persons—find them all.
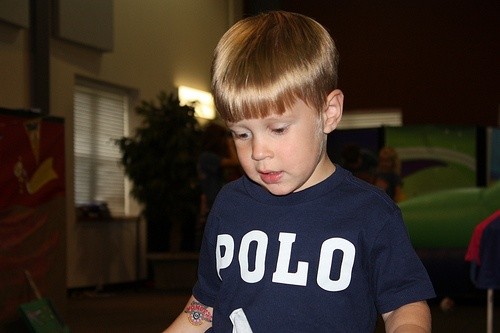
[339,141,404,203]
[441,210,500,313]
[162,11,437,333]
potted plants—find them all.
[112,91,231,289]
